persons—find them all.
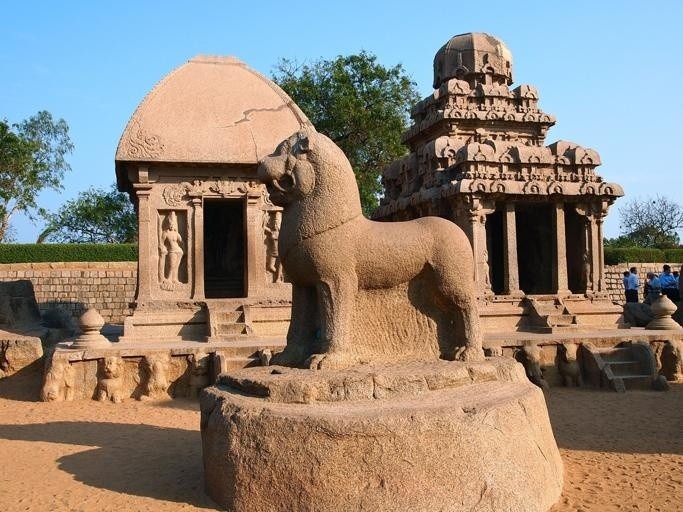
[623,264,683,304]
[159,212,183,281]
[261,211,283,284]
